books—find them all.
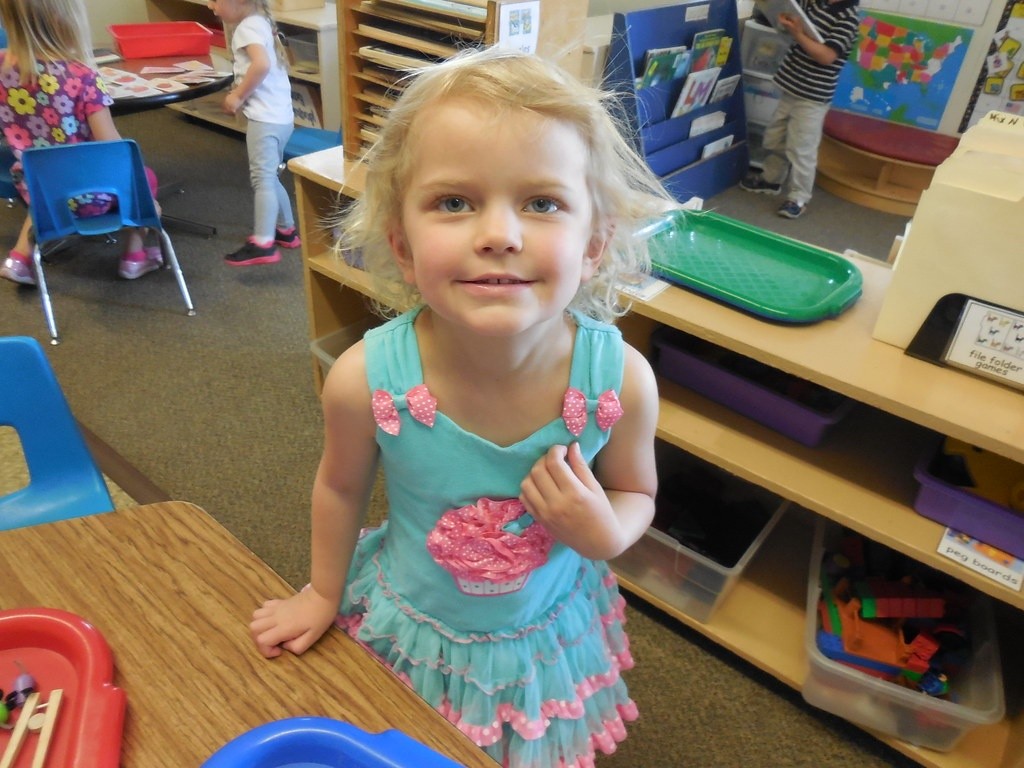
[755,0,826,47]
[352,1,497,166]
[633,29,741,166]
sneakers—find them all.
[224,235,281,266]
[778,200,806,218]
[739,174,782,195]
[0,257,37,285]
[119,247,164,279]
[276,225,301,247]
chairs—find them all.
[24,137,197,345]
[0,336,120,529]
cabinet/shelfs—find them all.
[145,1,339,157]
[288,0,1024,767]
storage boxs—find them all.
[801,513,1008,752]
[613,482,792,626]
[310,316,385,388]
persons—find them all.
[206,0,301,266]
[739,1,861,219]
[0,0,166,284]
[250,51,659,768]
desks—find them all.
[39,48,237,265]
[1,500,501,765]
